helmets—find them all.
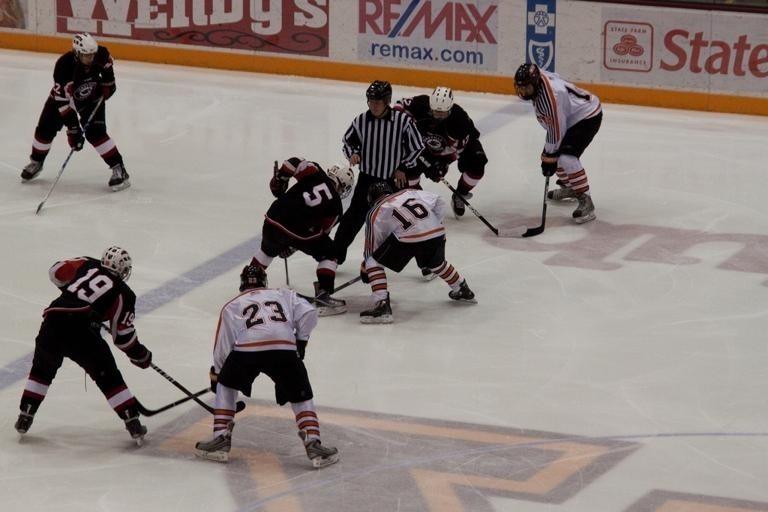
[239,266,267,291]
[429,86,454,121]
[366,80,391,104]
[514,63,540,101]
[327,166,355,198]
[366,182,393,207]
[100,246,131,281]
[72,33,98,54]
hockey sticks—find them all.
[522,172,549,237]
[274,160,314,303]
[103,324,246,416]
[131,386,212,417]
[36,94,104,213]
[419,157,527,238]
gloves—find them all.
[67,131,84,151]
[103,84,116,99]
[541,151,560,176]
[360,260,369,284]
[209,365,219,393]
[426,159,448,182]
[270,176,288,196]
[130,348,152,369]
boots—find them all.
[15,403,36,433]
[448,277,475,300]
[313,281,346,307]
[109,157,129,186]
[418,262,432,276]
[196,421,236,451]
[360,291,392,316]
[119,407,147,438]
[21,154,44,179]
[572,188,595,218]
[297,427,337,459]
[452,185,472,216]
[548,179,577,200]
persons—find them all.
[249,157,354,317]
[332,80,440,281]
[21,33,130,186]
[195,265,337,459]
[393,86,489,216]
[360,182,475,318]
[14,245,152,439]
[513,63,603,219]
